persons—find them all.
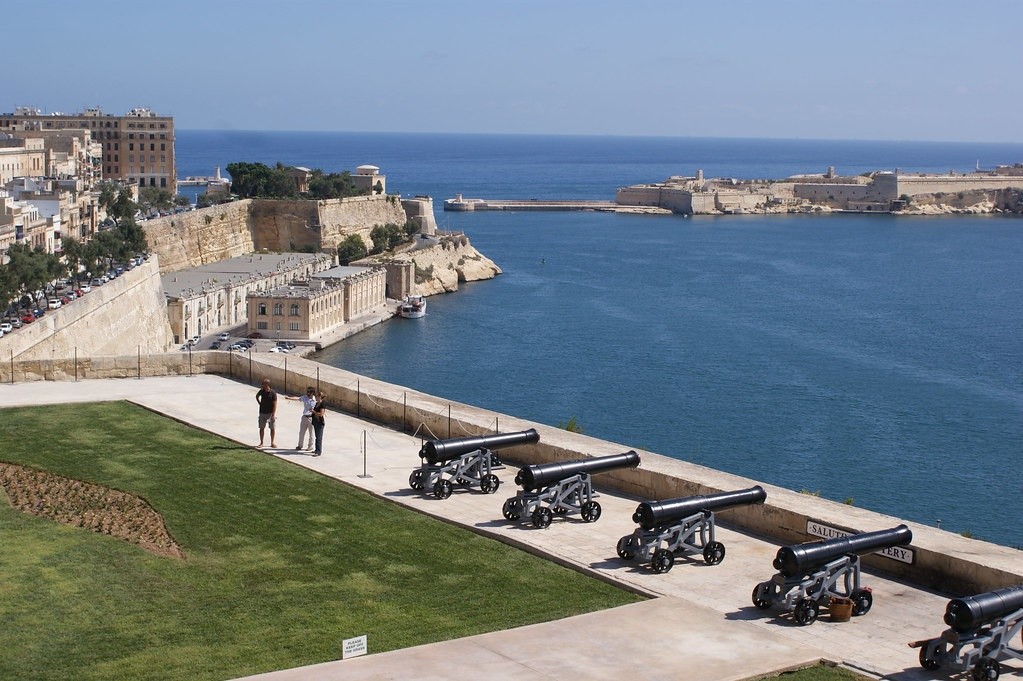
[256,379,278,448]
[285,387,326,457]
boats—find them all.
[400,292,428,318]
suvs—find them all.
[420,232,430,239]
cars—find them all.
[0,249,150,342]
[178,329,297,356]
[131,204,218,225]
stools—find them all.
[829,602,853,621]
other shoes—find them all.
[258,444,263,447]
[305,448,313,451]
[272,444,276,448]
[312,453,319,457]
[296,446,303,450]
[312,451,315,452]
